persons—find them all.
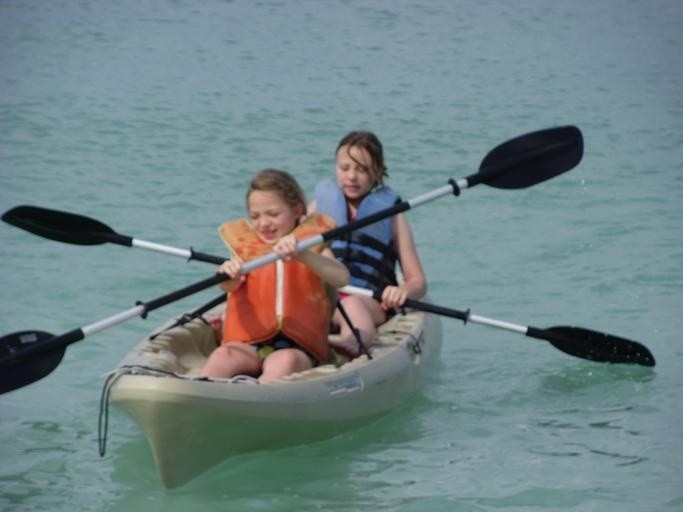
[201,169,350,383]
[307,131,426,358]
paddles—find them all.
[0,124,585,397]
[0,204,657,368]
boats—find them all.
[101,271,431,489]
